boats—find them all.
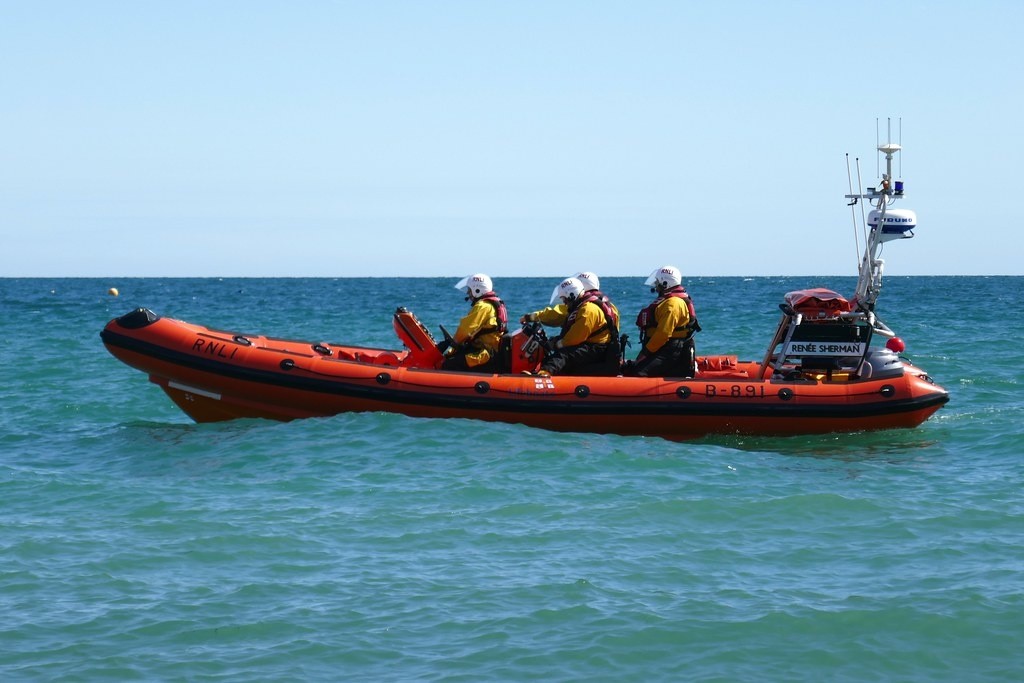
[101,117,950,447]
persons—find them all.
[520,272,620,331]
[439,273,508,372]
[521,278,620,377]
[620,264,698,378]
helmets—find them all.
[559,277,584,301]
[467,273,493,297]
[578,271,600,291]
[656,265,682,289]
